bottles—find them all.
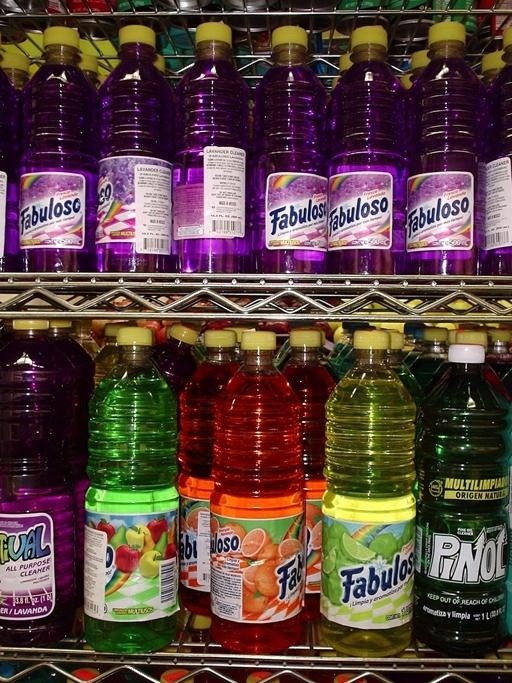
[3,2,512,308]
[0,293,512,678]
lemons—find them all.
[320,519,414,608]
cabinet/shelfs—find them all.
[1,0,511,683]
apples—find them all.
[96,516,177,580]
